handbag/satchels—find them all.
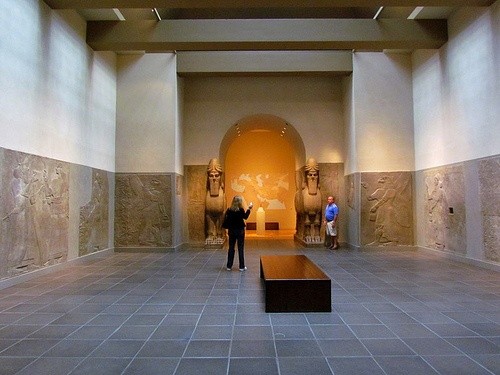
[222,208,229,229]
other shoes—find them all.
[239,267,248,272]
[227,266,233,270]
[329,244,339,250]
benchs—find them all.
[259,255,331,313]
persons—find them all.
[323,195,340,249]
[221,196,253,272]
